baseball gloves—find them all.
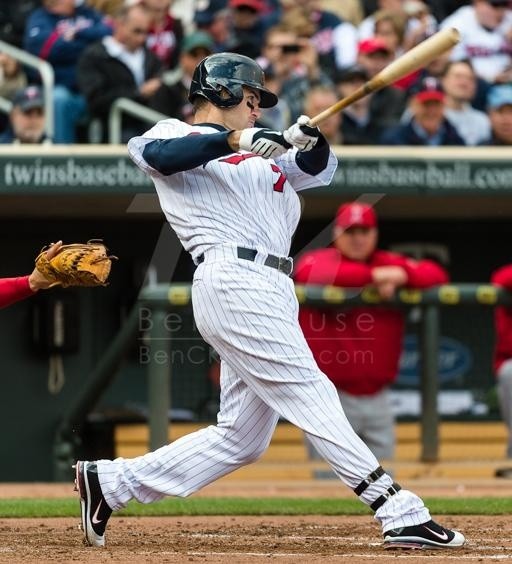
[34,238,118,289]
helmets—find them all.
[188,50,278,111]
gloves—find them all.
[239,126,292,159]
[284,114,320,153]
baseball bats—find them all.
[262,28,460,158]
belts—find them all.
[196,246,294,276]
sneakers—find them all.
[383,521,465,550]
[73,458,113,547]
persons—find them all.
[483,264,512,482]
[293,200,449,485]
[71,50,473,551]
[1,0,512,152]
[0,239,117,308]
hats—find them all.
[335,201,376,229]
[487,82,512,111]
[359,37,395,56]
[15,85,48,111]
[414,76,445,104]
